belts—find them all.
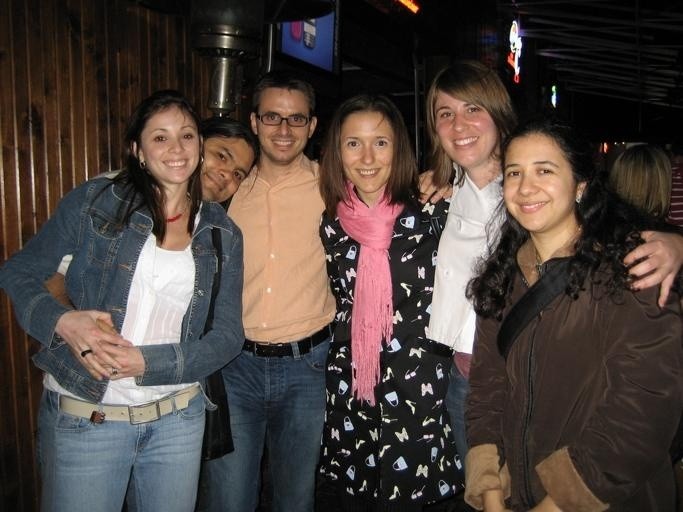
[57,384,201,424]
[243,319,339,357]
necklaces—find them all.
[162,196,191,222]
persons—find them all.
[0,89,243,511]
[463,119,682,511]
[424,60,682,511]
[39,115,261,310]
[608,143,671,220]
[312,90,475,509]
[201,69,456,509]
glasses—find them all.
[255,111,313,128]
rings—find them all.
[80,348,92,358]
[111,366,117,377]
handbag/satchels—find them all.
[200,370,235,462]
